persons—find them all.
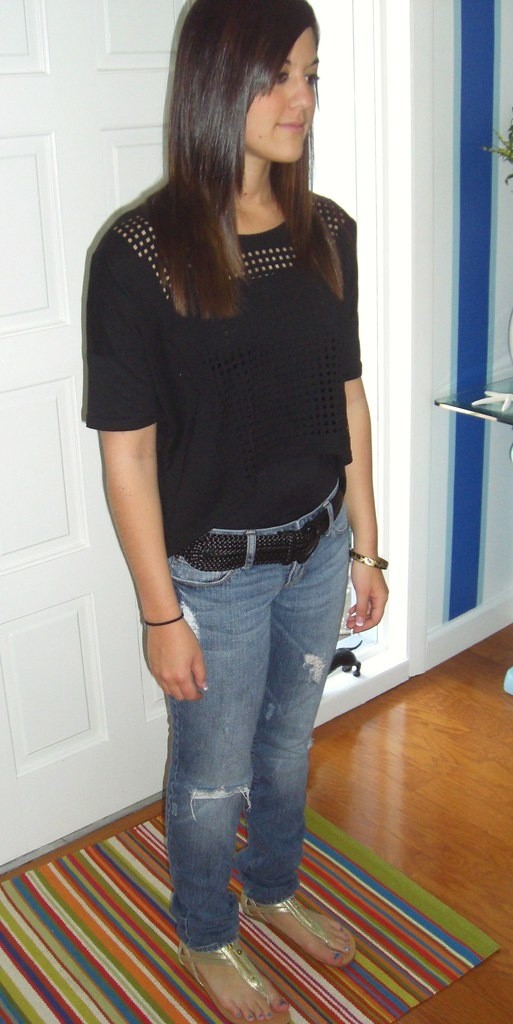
[85,0,389,1024]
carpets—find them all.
[0,799,501,1024]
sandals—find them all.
[178,936,291,1024]
[242,885,356,967]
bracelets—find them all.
[144,612,184,627]
[349,549,388,570]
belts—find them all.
[174,468,348,573]
